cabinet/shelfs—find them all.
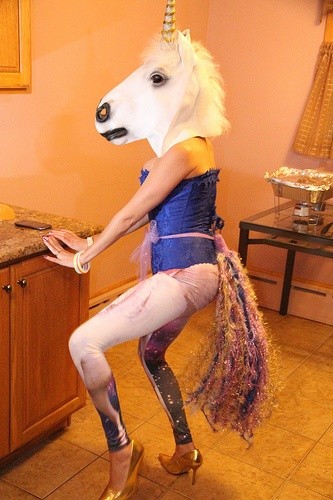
[0,201,105,468]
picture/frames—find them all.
[0,0,32,89]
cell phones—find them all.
[15,220,52,230]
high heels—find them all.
[157,446,205,488]
[94,436,148,500]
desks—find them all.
[237,200,333,315]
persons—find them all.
[43,29,231,500]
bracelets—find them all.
[73,251,90,274]
[87,237,93,246]
